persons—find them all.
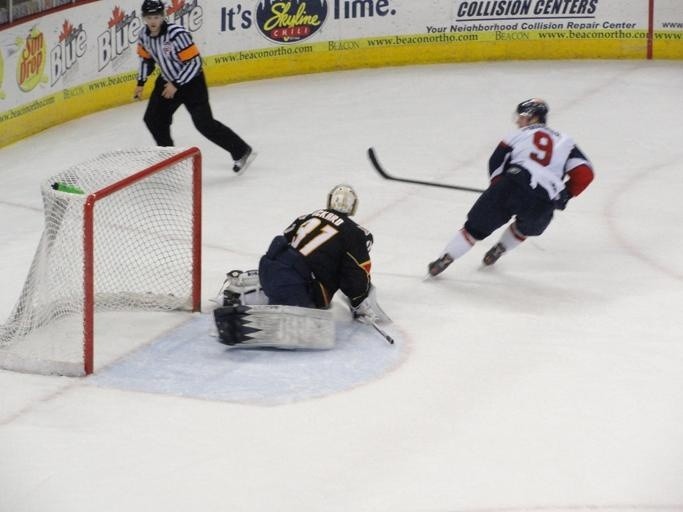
[134,0,252,172]
[428,97,594,276]
[213,182,378,350]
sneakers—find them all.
[232,146,252,173]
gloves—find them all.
[552,187,571,210]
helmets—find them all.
[141,0,164,32]
[326,183,359,217]
[515,97,547,128]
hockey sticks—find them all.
[368,149,487,194]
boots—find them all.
[428,253,453,277]
[483,242,506,265]
